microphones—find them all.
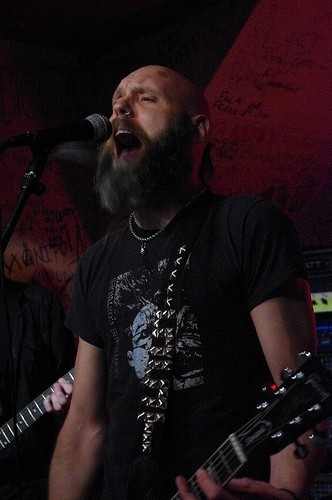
[0,114,113,150]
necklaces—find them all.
[126,187,205,258]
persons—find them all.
[42,63,328,500]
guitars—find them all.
[172,349,331,500]
